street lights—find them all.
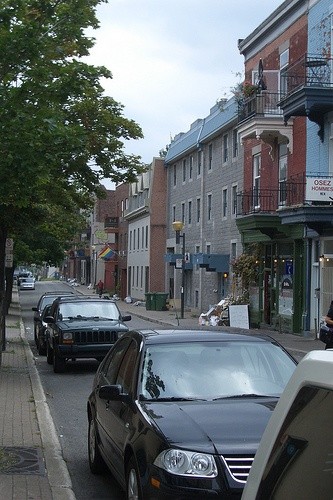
[91,245,97,291]
[172,221,186,319]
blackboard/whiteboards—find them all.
[228,304,250,330]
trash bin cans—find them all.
[144,292,156,311]
[95,284,103,294]
[156,292,169,312]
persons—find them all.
[325,300,333,350]
[98,280,104,296]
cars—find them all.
[13,271,37,291]
[31,291,75,357]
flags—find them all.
[98,246,115,261]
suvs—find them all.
[86,325,299,500]
[239,348,333,500]
[43,294,132,373]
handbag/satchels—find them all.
[319,325,333,345]
